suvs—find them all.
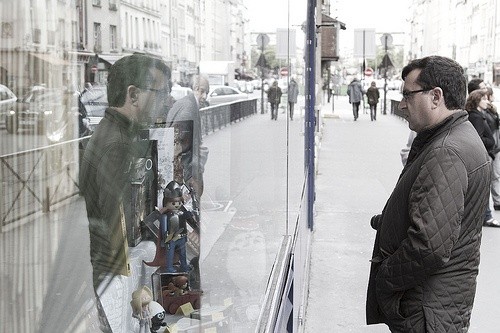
[6,84,88,135]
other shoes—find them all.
[485,218,500,227]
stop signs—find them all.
[365,68,373,77]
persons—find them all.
[347,78,364,121]
[267,80,282,120]
[366,56,492,333]
[80,53,176,332]
[483,87,500,130]
[465,90,500,228]
[366,82,380,121]
[166,75,209,201]
[288,77,299,121]
[468,79,500,211]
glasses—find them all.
[403,87,433,101]
[140,86,168,99]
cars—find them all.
[78,86,111,132]
[0,85,19,126]
[169,83,251,111]
